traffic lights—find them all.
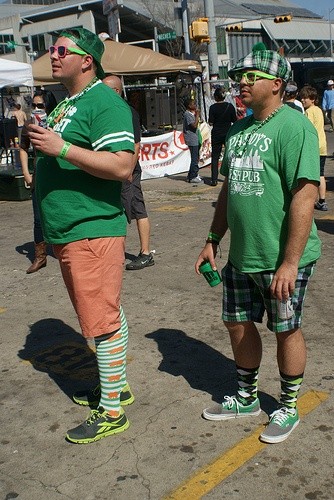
[273,14,292,23]
[189,20,209,40]
[225,22,242,32]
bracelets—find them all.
[205,231,223,246]
[58,142,71,159]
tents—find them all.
[30,34,203,122]
[0,58,34,99]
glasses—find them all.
[233,70,277,86]
[31,103,47,108]
[49,45,87,58]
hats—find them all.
[59,28,106,79]
[227,42,292,82]
[285,84,297,92]
[327,80,334,85]
[213,87,226,102]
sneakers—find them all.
[65,404,129,443]
[203,394,261,421]
[73,381,135,407]
[125,249,156,269]
[259,405,300,444]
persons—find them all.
[19,90,58,274]
[283,78,334,211]
[182,99,204,184]
[0,98,27,145]
[191,42,321,444]
[203,83,252,186]
[26,27,135,444]
[103,75,155,270]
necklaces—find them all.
[55,77,99,123]
[235,104,284,156]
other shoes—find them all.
[314,201,328,211]
[186,173,207,185]
[209,179,219,187]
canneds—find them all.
[30,109,47,130]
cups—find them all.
[199,260,221,288]
[31,130,48,157]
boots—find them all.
[26,241,47,273]
